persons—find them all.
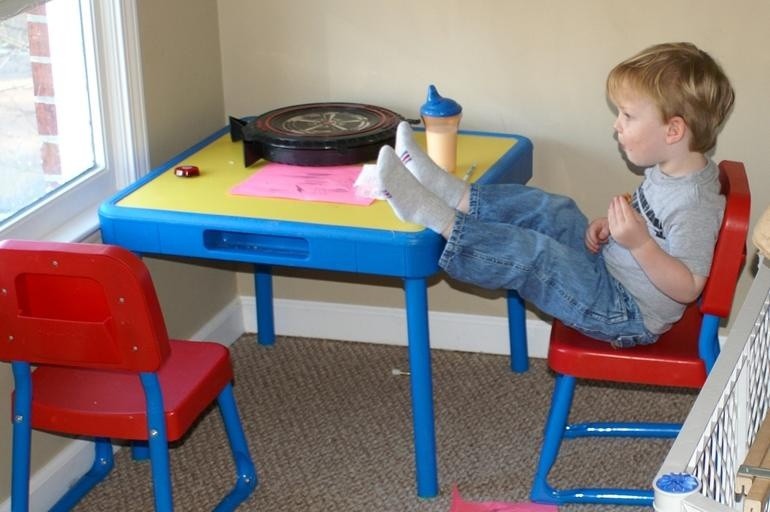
[373,37,738,352]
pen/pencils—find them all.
[463,165,476,182]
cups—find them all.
[419,84,463,173]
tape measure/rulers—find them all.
[175,165,199,177]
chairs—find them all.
[1,240,257,512]
[529,160,752,506]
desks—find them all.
[98,115,534,499]
